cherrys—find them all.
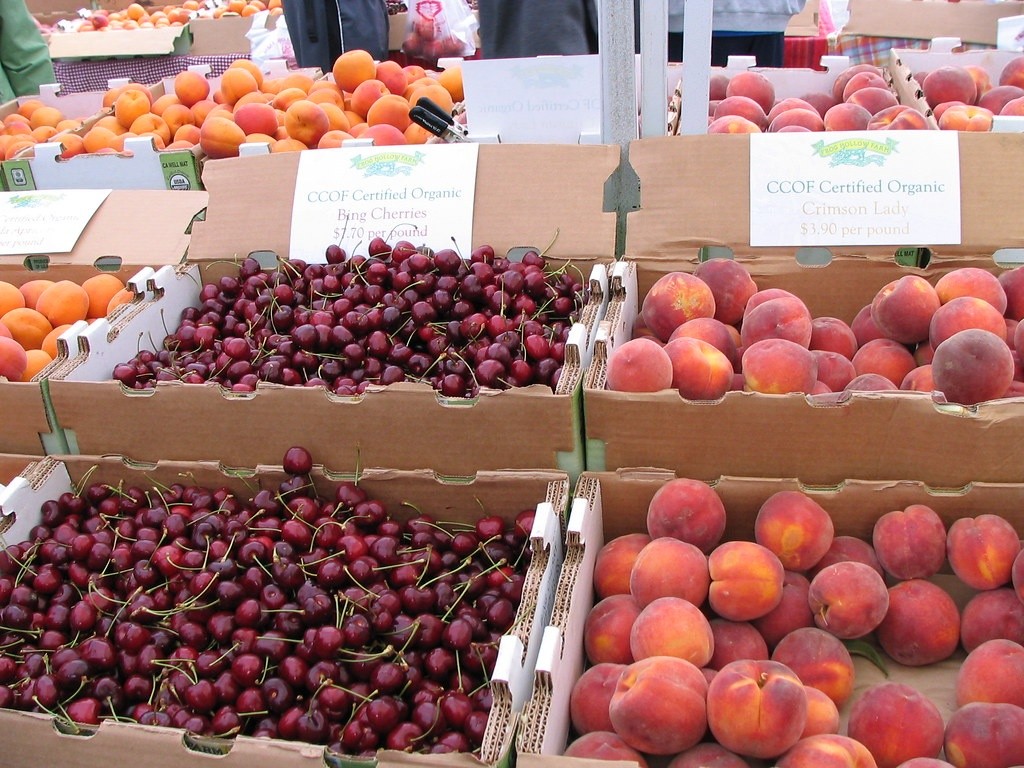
[111,210,588,397]
[0,445,535,760]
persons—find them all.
[475,0,598,59]
[0,0,58,105]
[281,0,390,75]
[667,0,806,68]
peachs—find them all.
[77,0,208,30]
[560,475,1024,768]
[197,0,284,19]
[603,258,1024,404]
[45,49,465,159]
[0,272,134,383]
[0,98,93,162]
[401,17,464,60]
[912,55,1024,132]
[708,63,930,134]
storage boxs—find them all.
[841,0,1024,46]
[1,60,323,191]
[460,54,684,143]
[583,130,1024,490]
[46,144,620,492]
[0,77,133,191]
[882,36,1024,131]
[0,188,208,455]
[0,454,565,768]
[25,1,277,65]
[516,465,1024,768]
